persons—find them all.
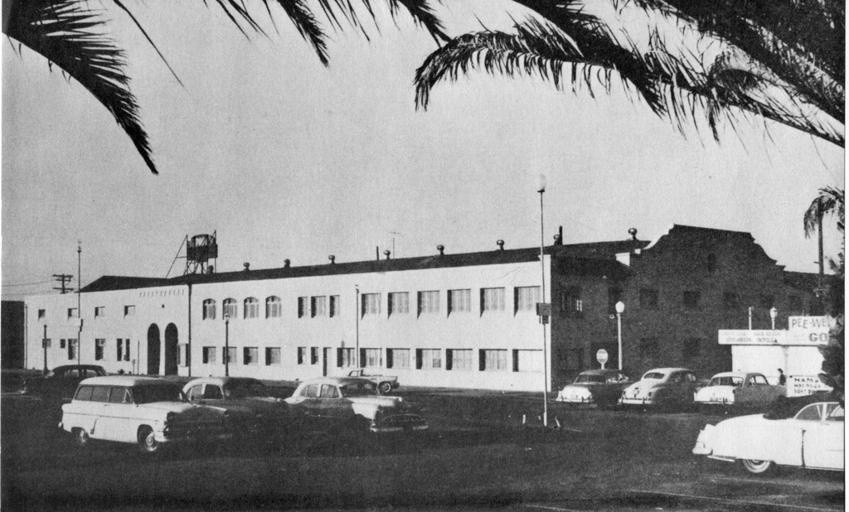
[775,367,786,387]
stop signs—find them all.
[595,348,609,366]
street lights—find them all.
[768,306,779,330]
[73,236,84,367]
[532,169,552,430]
[223,311,230,376]
[614,299,626,370]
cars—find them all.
[615,365,700,414]
[689,398,845,482]
[689,369,790,415]
[551,367,633,411]
